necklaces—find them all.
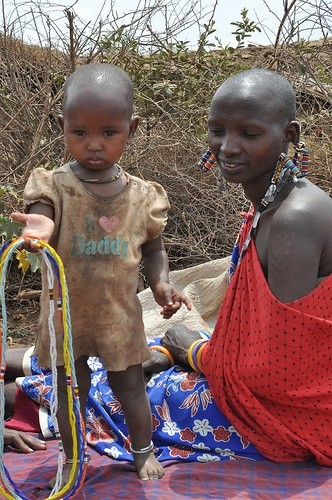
[72,163,125,185]
[235,176,278,267]
[0,232,90,500]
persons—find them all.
[2,426,48,455]
[8,62,193,491]
[0,67,332,470]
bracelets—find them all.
[186,338,210,375]
[198,328,217,343]
[148,344,175,367]
